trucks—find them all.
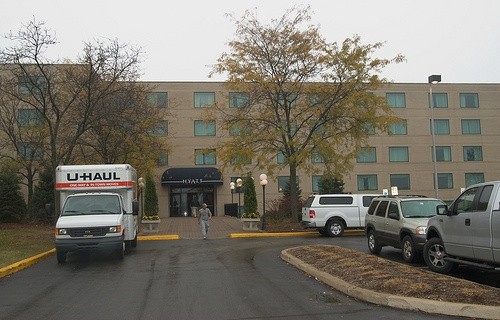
[55,164,138,264]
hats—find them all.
[202,204,207,208]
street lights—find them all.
[429,75,441,199]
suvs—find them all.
[301,193,388,237]
[423,181,500,275]
[365,194,449,263]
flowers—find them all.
[241,213,257,219]
[143,215,159,220]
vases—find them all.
[141,220,161,235]
[242,218,260,231]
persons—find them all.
[190,197,200,218]
[198,203,212,240]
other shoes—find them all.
[204,237,206,239]
